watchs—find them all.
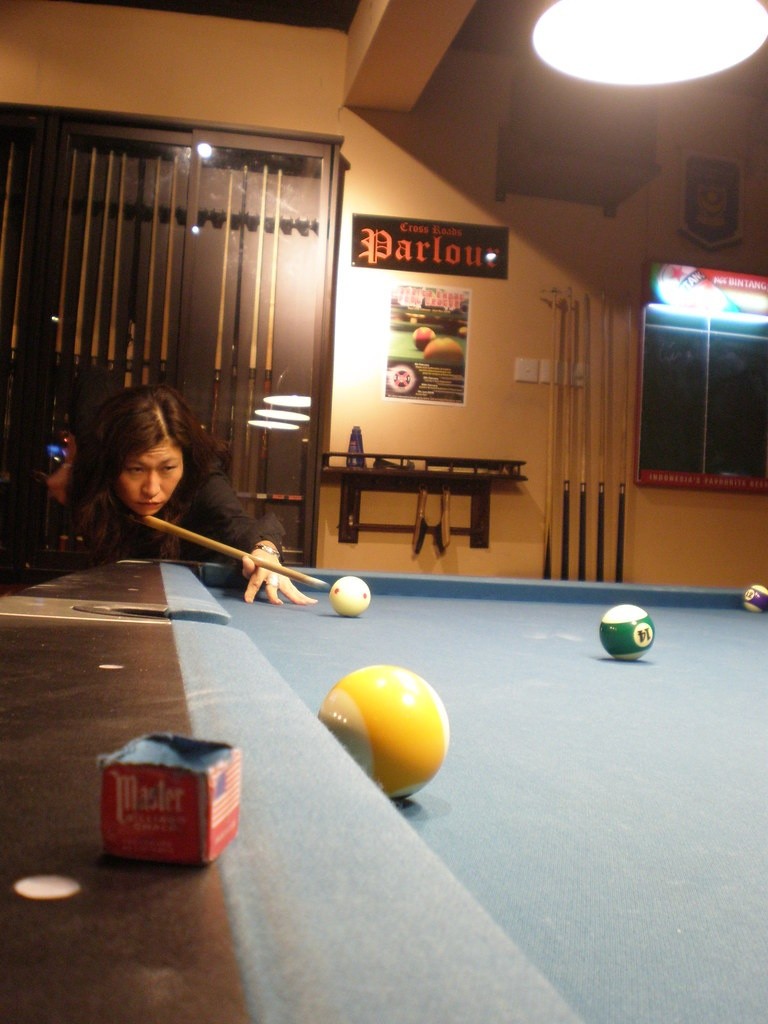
[249,542,280,560]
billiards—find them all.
[312,663,452,802]
[598,602,659,663]
[328,574,373,618]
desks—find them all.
[0,555,768,1024]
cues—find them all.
[540,284,636,582]
[0,138,287,583]
[29,470,332,593]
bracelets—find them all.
[61,464,72,472]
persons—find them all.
[46,383,319,606]
